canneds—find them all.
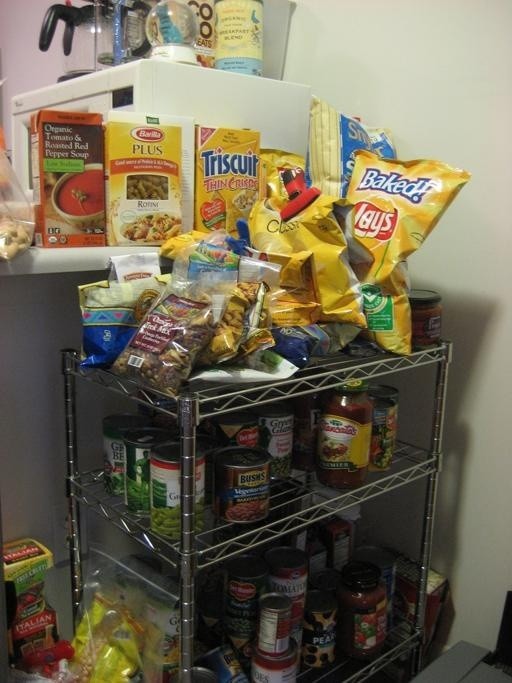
[294,385,322,471]
[368,384,399,471]
[257,409,295,478]
[115,545,387,683]
[214,446,273,523]
[408,288,443,345]
[150,442,205,539]
[318,375,373,488]
[212,416,258,448]
[122,426,172,519]
[104,415,143,498]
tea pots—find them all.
[39,0,157,84]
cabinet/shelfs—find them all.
[59,337,457,683]
